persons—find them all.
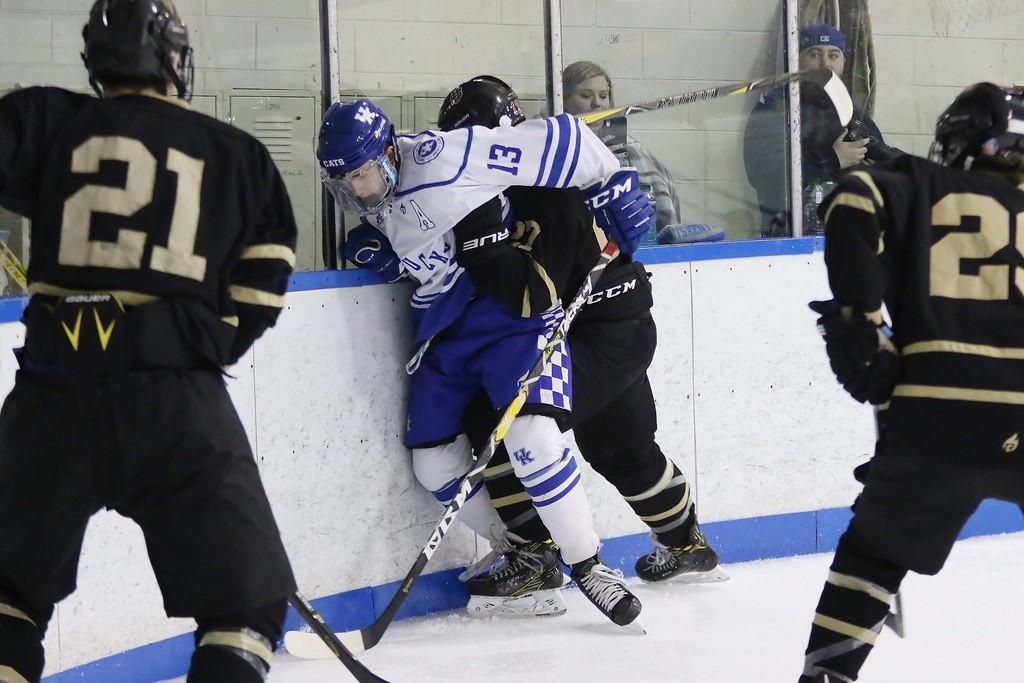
[560,64,680,239]
[439,75,729,617]
[741,25,885,237]
[0,0,299,683]
[799,78,1024,682]
[313,98,655,636]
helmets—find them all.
[80,0,195,102]
[928,82,1024,192]
[437,75,526,132]
[316,99,399,217]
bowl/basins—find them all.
[0,296,31,323]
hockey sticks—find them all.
[0,240,390,683]
[883,401,907,641]
[280,236,622,662]
[576,65,858,129]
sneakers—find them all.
[466,534,578,618]
[635,503,730,584]
[570,554,648,635]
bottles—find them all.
[803,176,824,236]
[637,183,658,246]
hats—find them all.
[799,24,848,58]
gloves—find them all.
[579,167,656,256]
[808,298,899,405]
[340,223,410,283]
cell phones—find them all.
[603,116,628,153]
